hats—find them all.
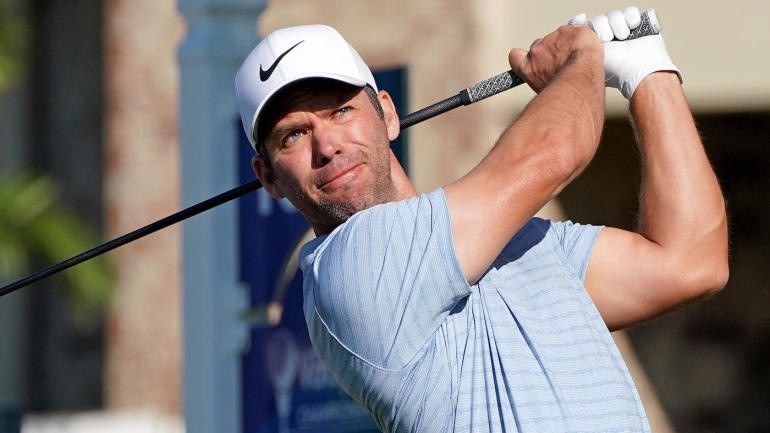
[235,23,379,154]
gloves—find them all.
[568,7,683,100]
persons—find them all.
[233,7,730,433]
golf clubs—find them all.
[0,8,663,298]
